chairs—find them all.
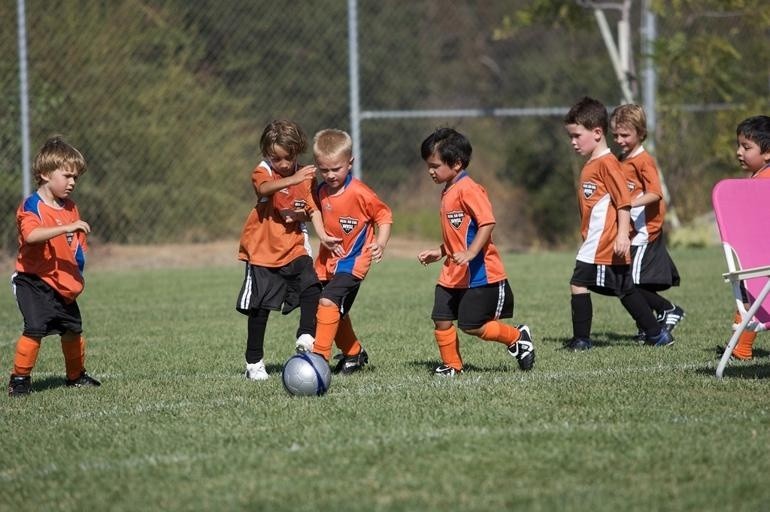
[712,180,770,378]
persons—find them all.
[236,121,346,380]
[8,136,102,395]
[715,115,770,360]
[279,128,394,374]
[609,104,686,341]
[417,127,536,378]
[555,96,675,352]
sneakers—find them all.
[296,334,315,353]
[432,365,464,379]
[65,368,100,387]
[506,324,534,370]
[564,338,591,352]
[9,374,30,395]
[636,303,685,346]
[247,359,269,381]
[333,345,368,375]
[717,333,734,353]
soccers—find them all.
[283,354,331,397]
